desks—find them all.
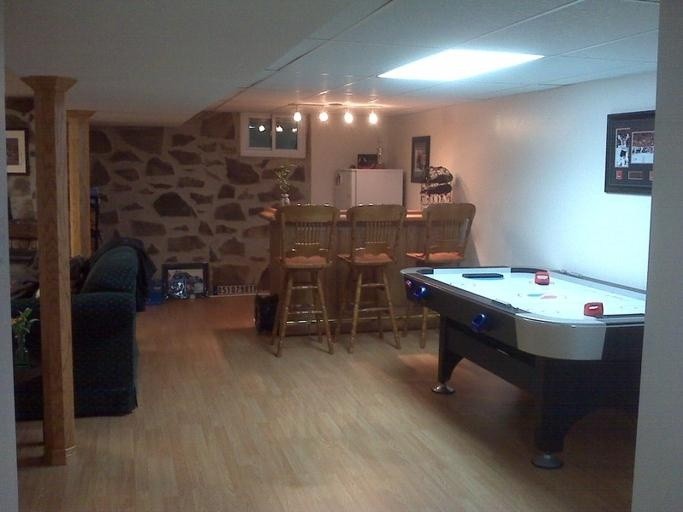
[399,265,646,468]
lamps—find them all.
[287,104,378,124]
[259,121,298,134]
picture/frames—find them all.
[604,110,655,195]
[411,136,430,182]
[5,128,30,175]
[161,262,209,302]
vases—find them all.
[15,337,31,368]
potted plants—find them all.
[273,165,291,206]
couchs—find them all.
[10,235,141,421]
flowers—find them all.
[11,307,41,337]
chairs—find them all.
[333,204,408,353]
[270,204,341,357]
[401,202,476,349]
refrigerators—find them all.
[333,168,404,211]
